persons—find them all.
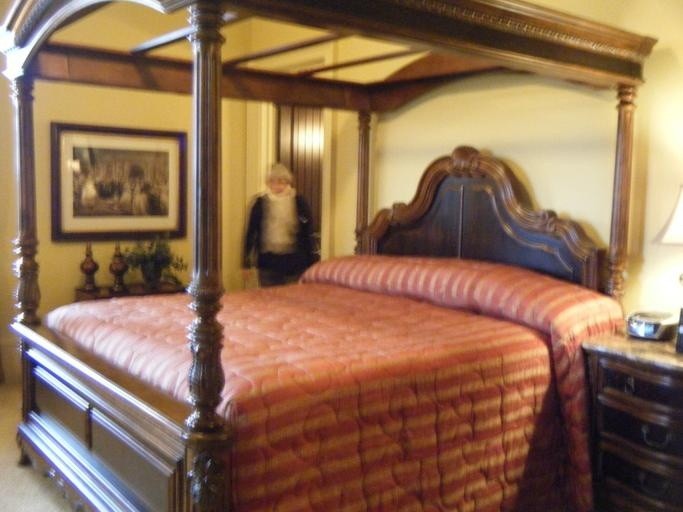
[240,164,317,288]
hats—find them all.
[266,163,292,180]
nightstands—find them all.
[588,332,683,512]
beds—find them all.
[23,150,605,512]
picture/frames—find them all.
[48,120,191,242]
